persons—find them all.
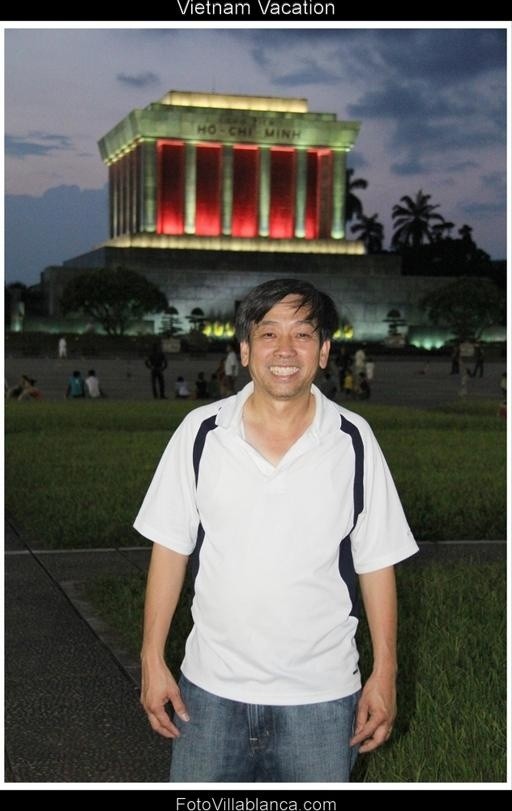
[130,274,421,782]
[445,344,460,375]
[365,357,377,381]
[343,367,354,399]
[353,344,369,376]
[175,376,192,399]
[6,375,43,400]
[318,373,337,400]
[145,339,169,400]
[209,341,240,400]
[471,340,487,377]
[65,370,85,398]
[85,370,107,398]
[58,335,68,361]
[354,372,371,400]
[334,344,352,392]
[194,371,210,399]
